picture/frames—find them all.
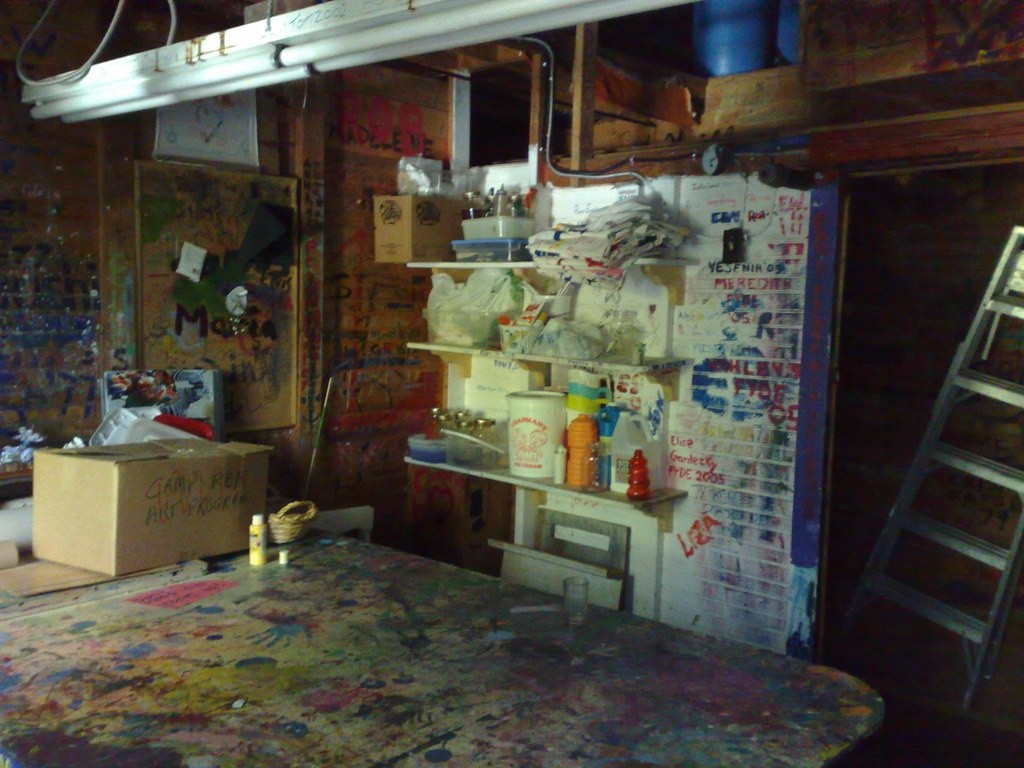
[133,159,299,435]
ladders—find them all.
[834,227,1024,722]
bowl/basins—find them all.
[449,217,537,263]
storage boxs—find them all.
[799,0,1024,126]
[451,240,534,263]
[373,194,484,263]
[700,61,804,136]
[459,215,535,239]
[566,115,701,154]
[33,441,275,579]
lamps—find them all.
[22,0,696,122]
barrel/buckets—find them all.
[506,392,566,479]
[692,0,800,76]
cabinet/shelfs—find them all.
[407,159,849,664]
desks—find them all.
[1,525,886,767]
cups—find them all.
[563,577,589,625]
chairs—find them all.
[309,506,373,544]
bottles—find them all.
[430,407,501,460]
[492,182,537,216]
[248,514,267,565]
[565,402,661,499]
[554,444,566,485]
[460,191,483,219]
